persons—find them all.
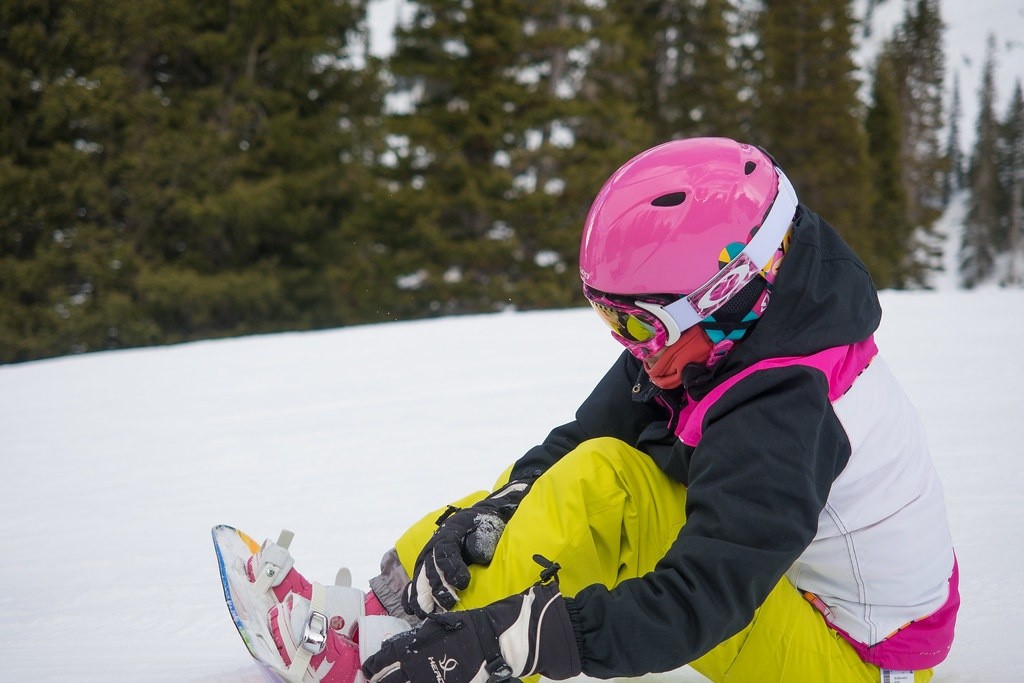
[212,136,962,683]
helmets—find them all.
[578,137,799,324]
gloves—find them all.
[361,554,581,683]
[401,479,535,621]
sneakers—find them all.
[212,524,412,683]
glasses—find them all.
[582,287,681,361]
